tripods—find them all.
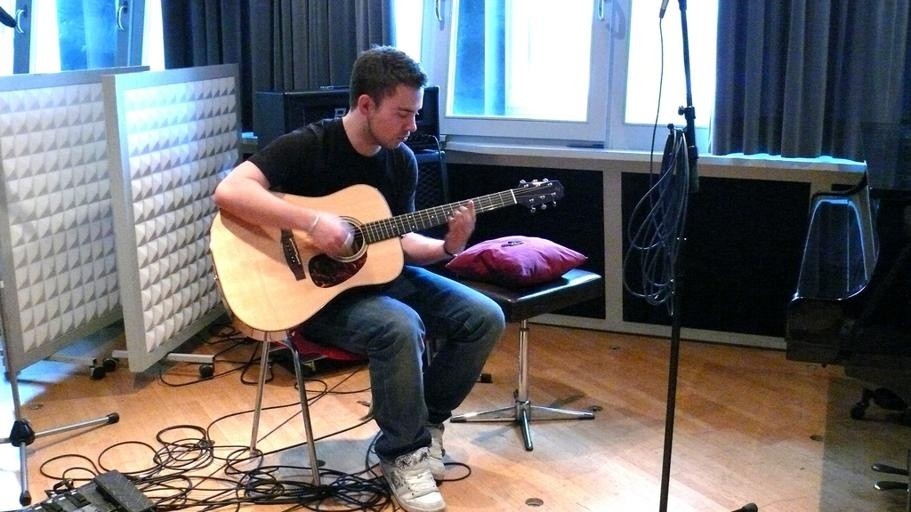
[0,287,119,507]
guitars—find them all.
[211,178,566,341]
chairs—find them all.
[450,262,602,452]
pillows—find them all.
[445,233,588,286]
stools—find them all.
[246,331,370,488]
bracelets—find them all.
[441,241,457,258]
[305,212,322,235]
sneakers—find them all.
[380,427,447,511]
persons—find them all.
[212,47,507,512]
